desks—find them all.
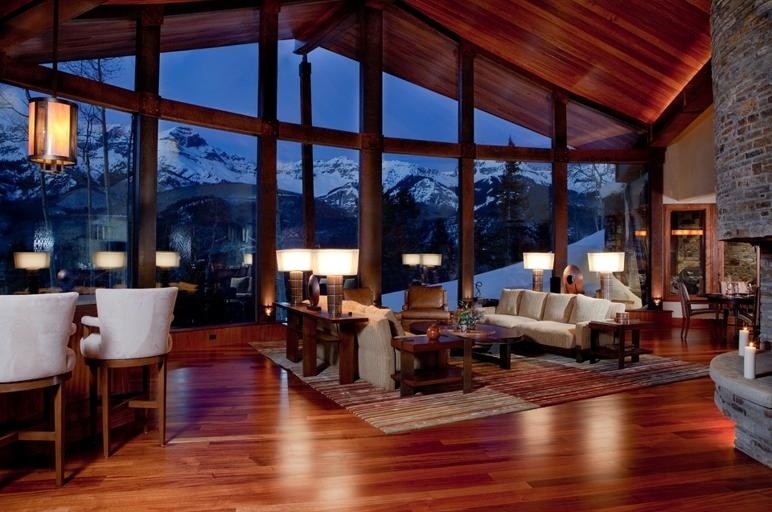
[626,309,673,340]
[278,302,368,384]
[707,292,756,332]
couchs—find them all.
[303,295,421,392]
[475,303,624,363]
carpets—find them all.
[248,338,710,434]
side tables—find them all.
[589,319,646,368]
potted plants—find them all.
[452,302,482,330]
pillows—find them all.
[231,277,249,292]
[342,301,405,336]
[496,288,610,324]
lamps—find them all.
[588,251,625,300]
[276,248,312,304]
[12,251,182,294]
[401,254,442,285]
[523,252,555,292]
[28,0,78,175]
[310,249,360,316]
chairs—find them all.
[677,282,722,340]
[80,287,178,457]
[401,285,451,333]
[0,291,79,487]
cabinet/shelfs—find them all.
[391,333,474,398]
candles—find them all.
[738,327,756,379]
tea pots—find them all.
[427,322,440,341]
